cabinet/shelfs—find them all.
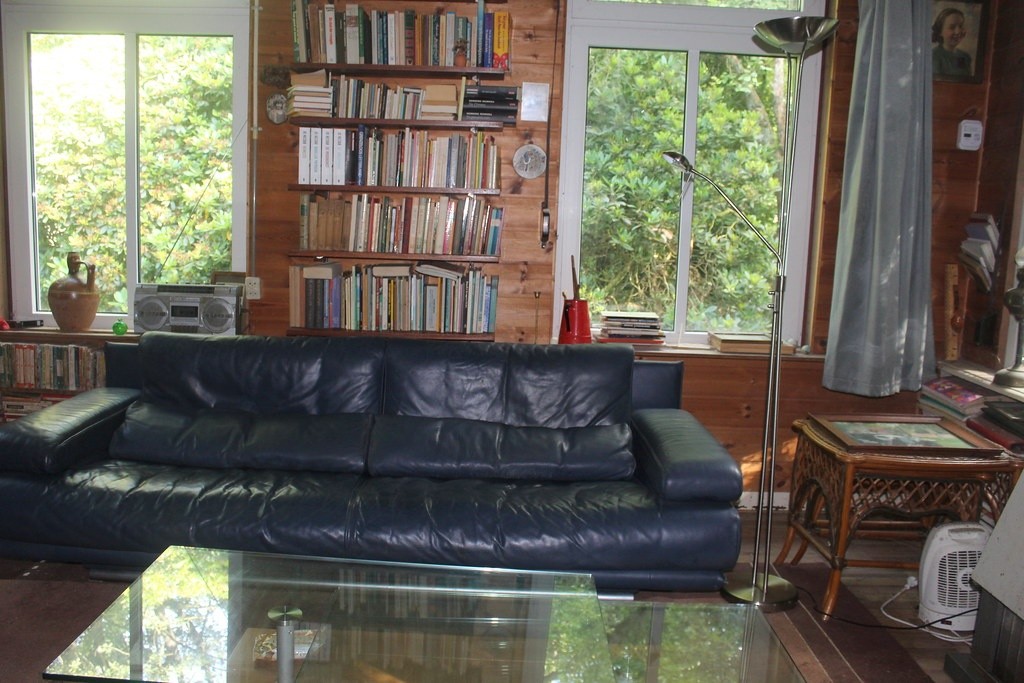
[0,329,139,427]
[243,0,569,335]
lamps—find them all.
[664,11,842,609]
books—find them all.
[955,211,1001,294]
[284,0,522,335]
[917,374,1024,452]
[0,342,105,422]
[709,337,796,355]
[601,312,665,338]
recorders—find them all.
[135,282,245,338]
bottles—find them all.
[112,317,127,335]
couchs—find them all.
[0,327,745,592]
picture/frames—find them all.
[930,0,994,85]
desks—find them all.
[45,539,809,683]
[779,408,1024,624]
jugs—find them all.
[559,299,592,344]
[48,252,99,331]
